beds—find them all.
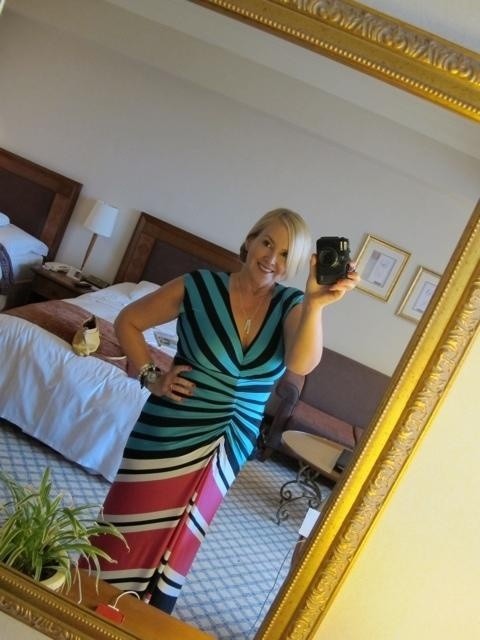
[1,210,247,486]
[0,146,85,312]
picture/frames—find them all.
[395,265,443,326]
[352,232,412,305]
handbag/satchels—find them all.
[71,314,102,358]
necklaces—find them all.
[236,272,265,335]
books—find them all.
[336,449,354,471]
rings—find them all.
[170,383,174,391]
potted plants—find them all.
[0,457,132,610]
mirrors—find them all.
[0,0,478,640]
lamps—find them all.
[64,197,122,282]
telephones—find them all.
[41,261,70,273]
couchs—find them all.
[257,343,393,485]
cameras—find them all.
[316,236,350,285]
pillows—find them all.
[0,209,11,228]
[128,279,163,302]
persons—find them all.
[77,207,363,616]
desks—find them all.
[46,562,219,640]
[287,507,322,567]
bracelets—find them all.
[138,362,161,389]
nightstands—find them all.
[20,262,104,308]
